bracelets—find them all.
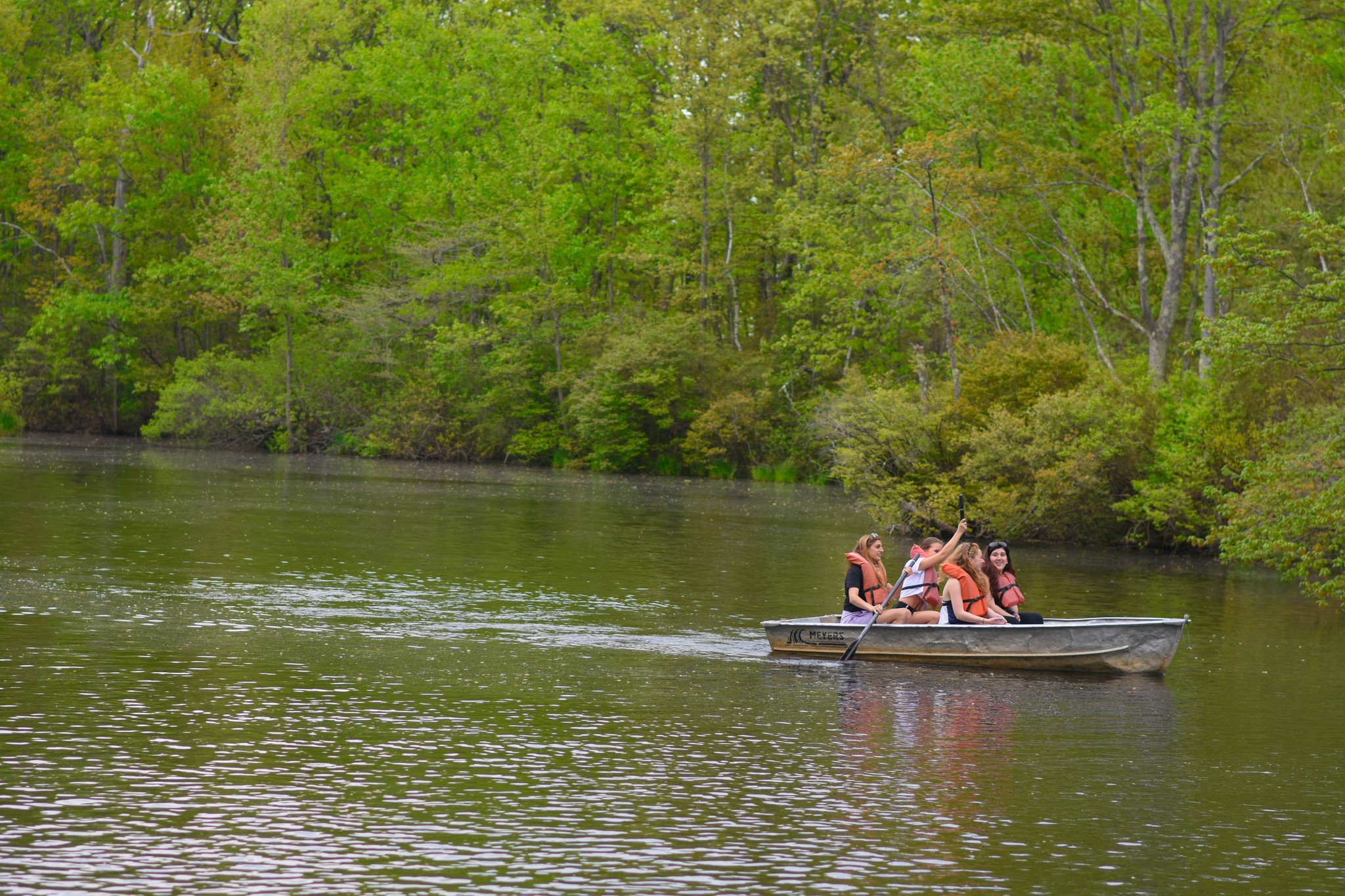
[978,616,981,623]
[982,618,986,624]
[1011,611,1018,616]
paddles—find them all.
[839,553,921,662]
[958,492,966,542]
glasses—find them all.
[869,533,879,539]
[968,542,972,554]
[989,542,1007,547]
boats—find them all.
[760,613,1192,674]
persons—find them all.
[937,543,1011,624]
[841,533,912,624]
[983,541,1043,625]
[892,518,967,623]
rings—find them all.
[878,607,881,609]
